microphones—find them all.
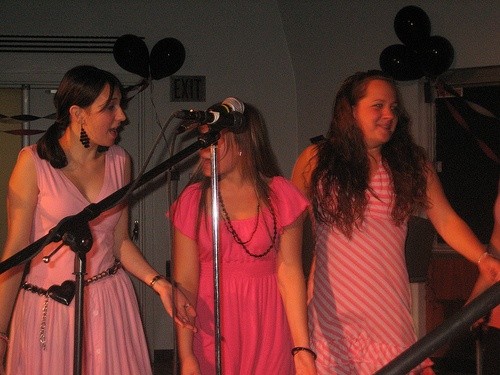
[174,109,252,133]
[176,95,245,137]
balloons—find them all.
[393,5,431,50]
[113,34,149,77]
[421,36,455,73]
[149,38,185,80]
[380,44,424,81]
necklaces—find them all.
[217,183,276,257]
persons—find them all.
[166,102,317,375]
[464,194,500,375]
[0,65,196,375]
[292,70,500,375]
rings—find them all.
[185,303,191,308]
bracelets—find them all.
[477,252,491,263]
[149,275,164,286]
[291,346,317,360]
